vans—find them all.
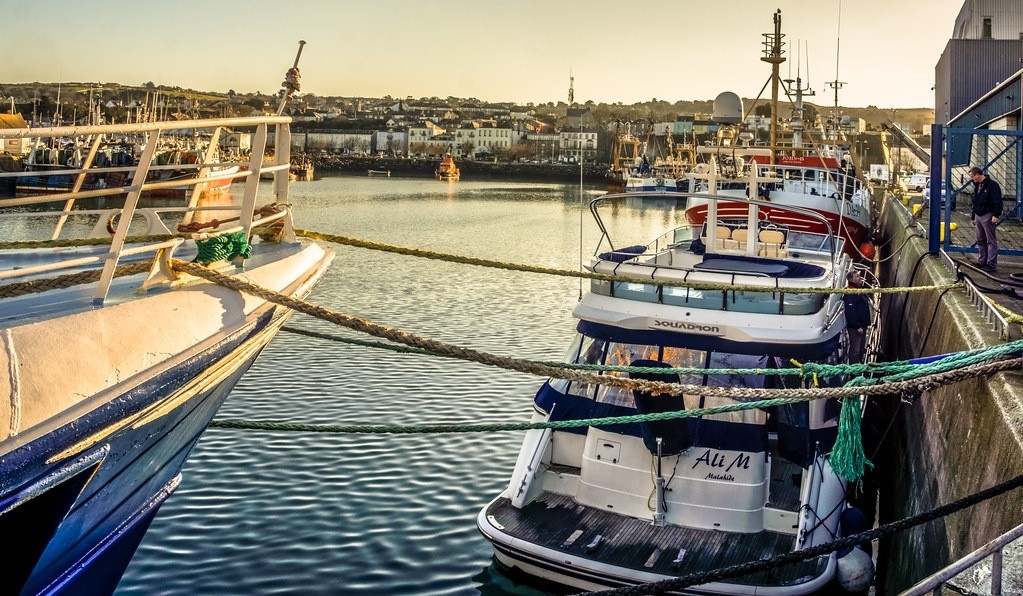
[899,173,929,192]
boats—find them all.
[0,40,336,596]
[435,155,460,181]
[477,0,882,596]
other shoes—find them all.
[971,262,985,268]
[986,266,997,273]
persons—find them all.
[968,167,1003,271]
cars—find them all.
[923,180,956,209]
